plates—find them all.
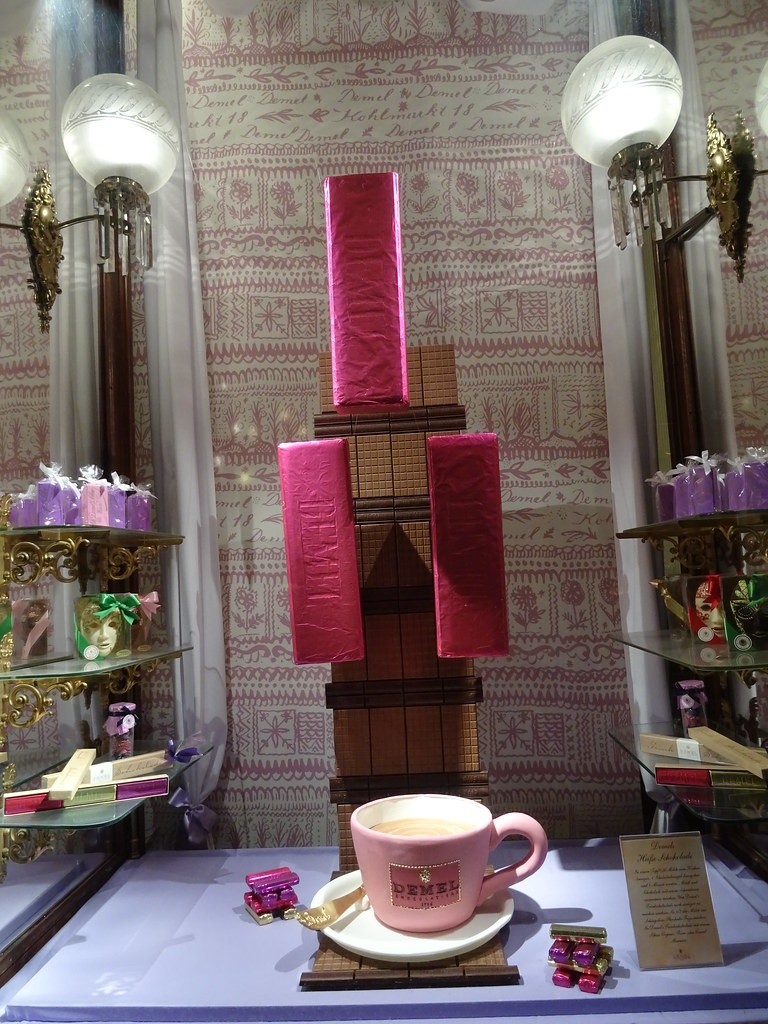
[311,869,515,961]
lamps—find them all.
[557,34,743,284]
[731,59,768,256]
[25,71,182,333]
[0,101,35,305]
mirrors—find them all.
[634,0,768,744]
[0,0,147,994]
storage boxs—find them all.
[719,572,768,652]
[680,574,727,645]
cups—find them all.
[349,794,548,932]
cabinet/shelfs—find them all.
[0,518,218,831]
[608,509,768,881]
